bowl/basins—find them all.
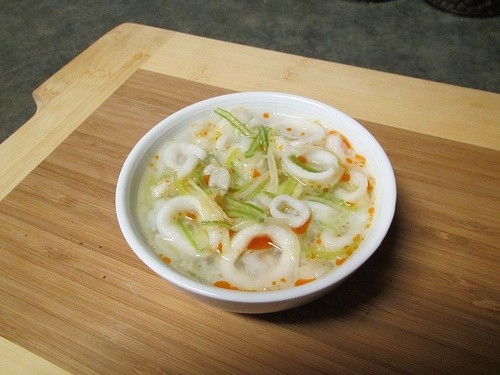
[117,90,398,315]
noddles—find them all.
[138,110,370,291]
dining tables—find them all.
[0,21,500,374]
